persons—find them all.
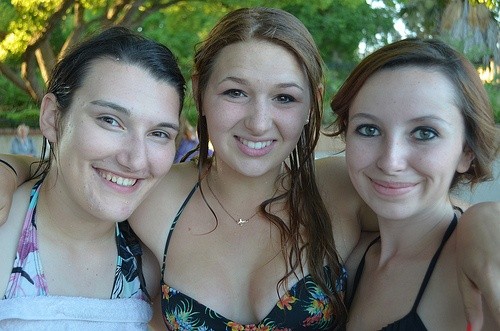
[320,38,500,331]
[10,124,36,155]
[0,25,186,331]
[174,122,215,164]
[0,6,474,331]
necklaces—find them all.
[206,176,279,225]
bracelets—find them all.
[0,159,17,176]
